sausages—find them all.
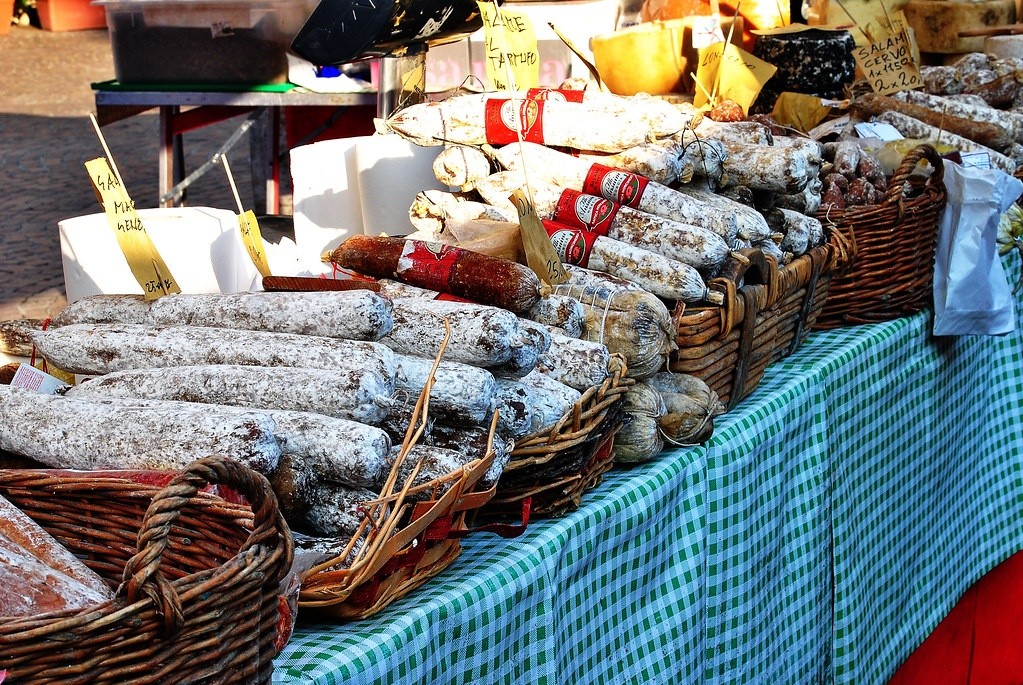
[0,50,1023,618]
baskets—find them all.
[299,317,500,619]
[668,224,857,410]
[0,455,293,685]
[480,350,636,522]
[809,144,946,330]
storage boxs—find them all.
[92,0,319,86]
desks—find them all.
[89,79,376,217]
[270,242,1022,685]
